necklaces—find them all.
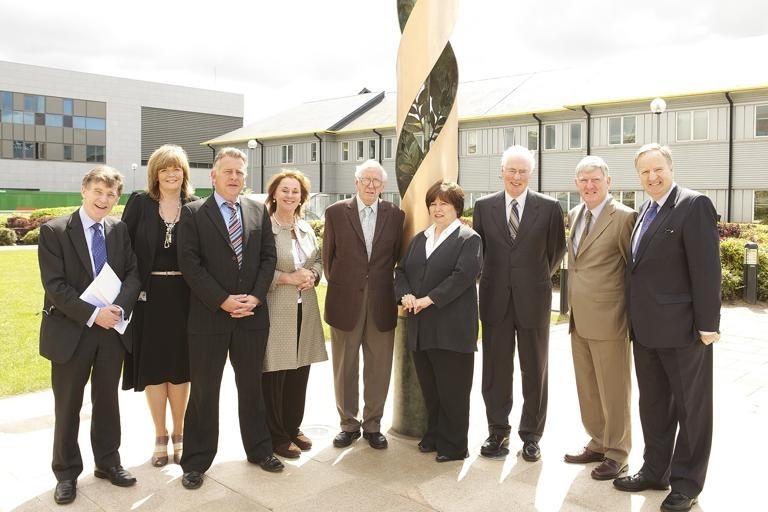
[157,200,181,250]
[271,211,297,233]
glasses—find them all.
[361,178,383,189]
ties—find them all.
[575,210,592,259]
[223,201,243,270]
[507,199,519,245]
[362,207,374,262]
[633,201,658,259]
[91,224,107,277]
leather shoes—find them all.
[151,429,169,466]
[54,479,77,504]
[420,445,469,462]
[564,447,604,463]
[171,434,183,464]
[333,430,361,448]
[522,440,541,462]
[182,471,203,489]
[247,435,312,472]
[613,473,668,492]
[591,458,629,480]
[481,434,510,455]
[94,464,136,487]
[363,431,388,449]
[660,490,698,512]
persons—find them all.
[564,156,638,481]
[395,181,484,462]
[322,160,405,450]
[120,145,203,466]
[614,142,722,508]
[176,146,285,490]
[474,144,566,461]
[38,166,140,505]
[260,167,324,459]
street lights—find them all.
[247,137,258,190]
[131,162,139,190]
[649,95,665,145]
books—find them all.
[78,262,134,335]
